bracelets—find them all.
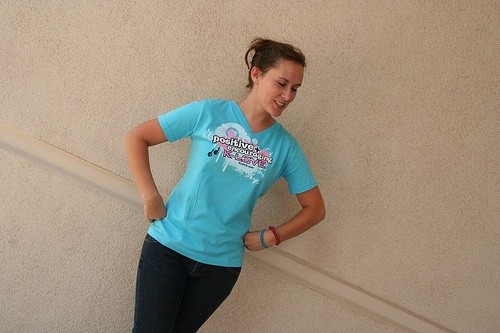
[258,230,270,248]
[268,226,282,246]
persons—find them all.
[125,37,328,333]
